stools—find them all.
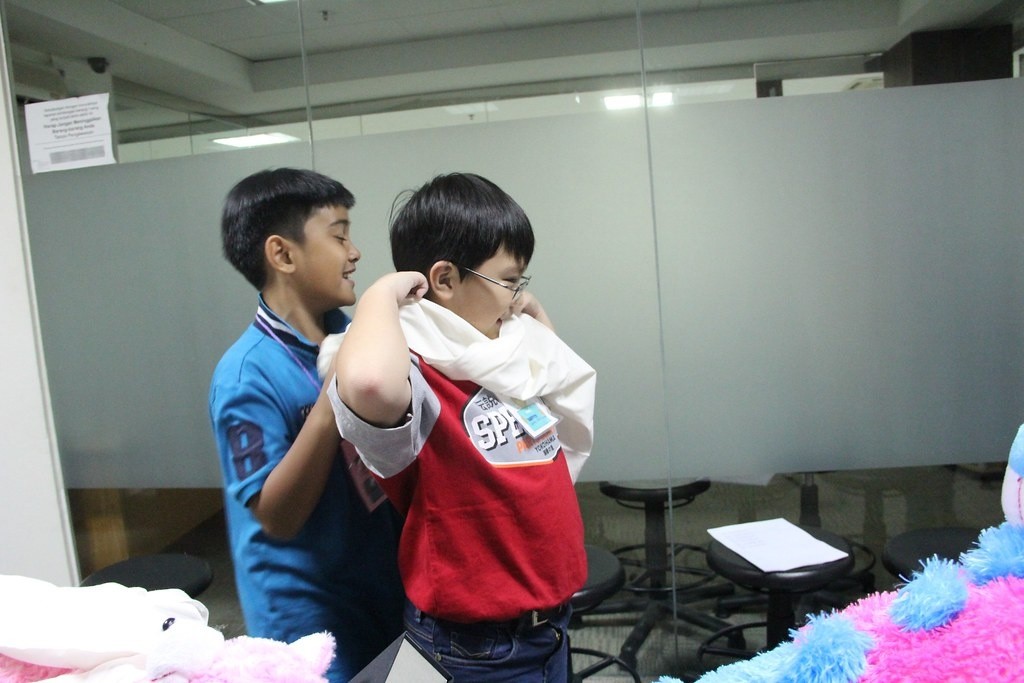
[78,552,216,601]
[568,545,641,683]
[569,475,746,670]
[696,526,854,674]
[882,526,992,583]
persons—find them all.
[208,168,404,683]
[326,172,597,683]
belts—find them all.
[474,605,567,627]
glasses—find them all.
[430,258,532,304]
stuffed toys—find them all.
[0,575,336,683]
[653,421,1024,683]
